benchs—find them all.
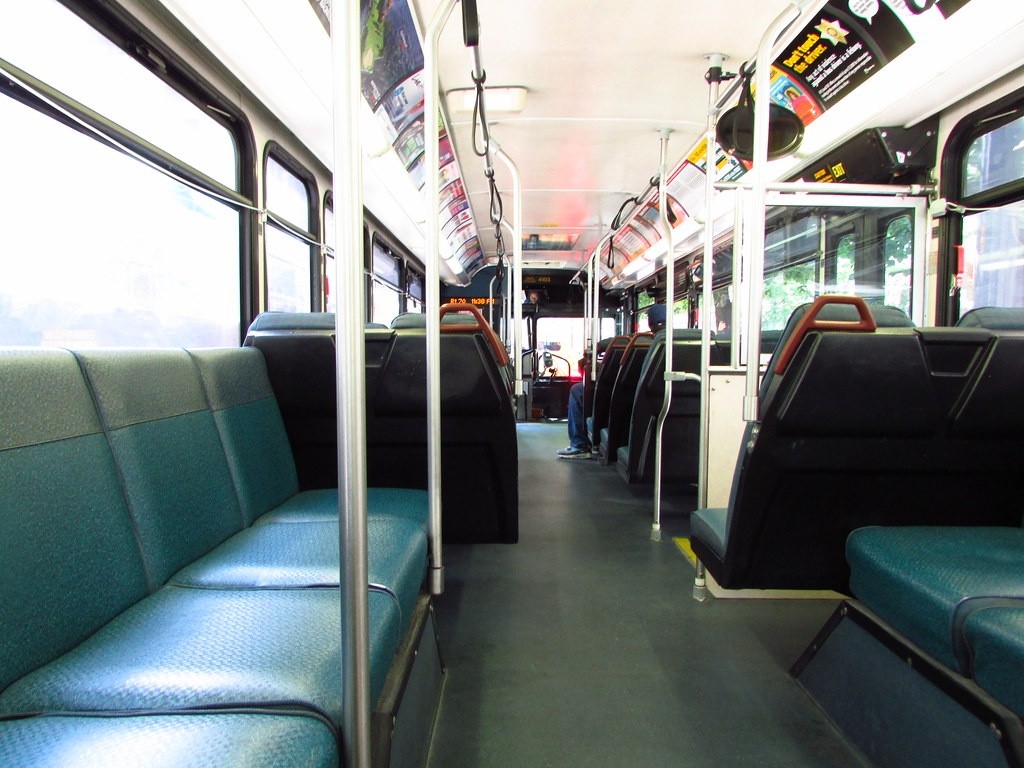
[586,294,1024,768]
[1,303,516,768]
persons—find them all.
[529,292,539,304]
[557,304,666,458]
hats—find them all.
[645,304,666,327]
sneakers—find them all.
[557,446,593,459]
